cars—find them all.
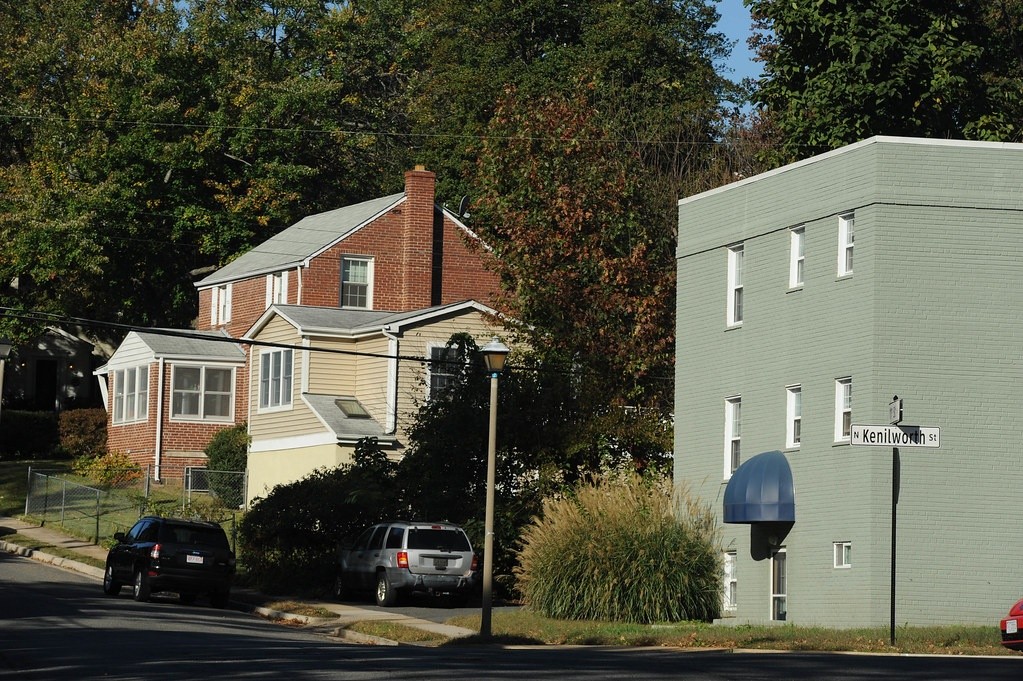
[1000,597,1023,650]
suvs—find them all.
[104,515,236,606]
[335,519,479,607]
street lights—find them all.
[478,335,510,636]
